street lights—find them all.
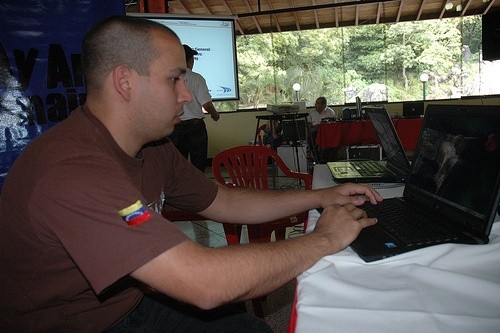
[293,83,301,101]
[420,73,429,100]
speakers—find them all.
[482,10,500,62]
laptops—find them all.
[327,108,411,184]
[316,97,500,262]
[403,101,424,119]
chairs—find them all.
[212,146,313,319]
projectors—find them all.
[267,104,299,113]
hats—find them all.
[182,43,199,55]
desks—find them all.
[287,160,500,333]
[315,118,424,160]
[247,113,319,190]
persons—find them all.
[0,15,382,332]
[170,44,220,173]
[307,97,337,125]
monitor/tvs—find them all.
[356,96,362,119]
